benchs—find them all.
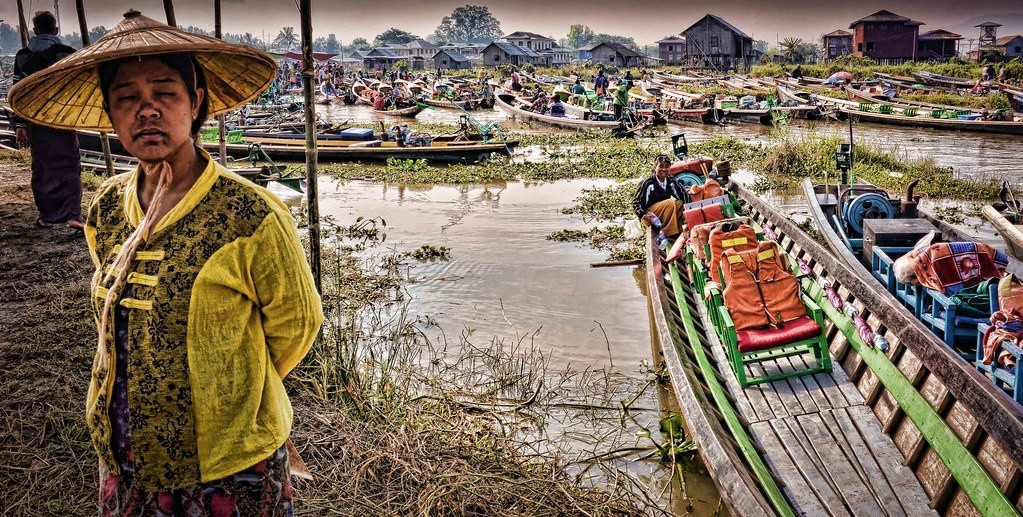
[873,104,894,115]
[922,109,944,119]
[446,140,477,145]
[948,110,972,119]
[901,106,919,116]
[853,102,872,111]
[351,140,383,147]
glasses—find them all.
[657,156,671,161]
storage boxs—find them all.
[340,126,373,141]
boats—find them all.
[77,89,519,201]
[644,156,1022,517]
[351,65,1022,134]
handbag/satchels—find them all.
[597,84,603,94]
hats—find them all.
[7,8,278,131]
[626,81,635,87]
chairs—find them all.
[574,94,605,110]
[871,242,1023,403]
[540,102,568,117]
[683,202,832,389]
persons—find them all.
[213,59,1006,140]
[632,154,692,245]
[6,7,326,517]
[12,11,86,232]
